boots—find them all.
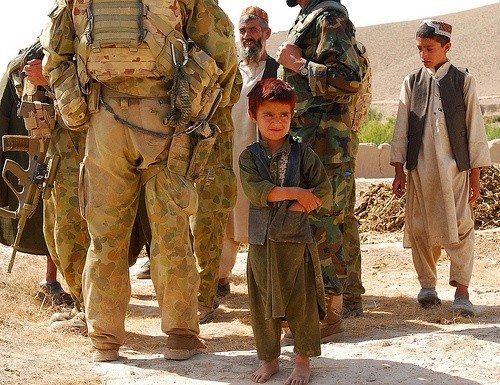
[282,295,345,345]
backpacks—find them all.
[286,1,380,133]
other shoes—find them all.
[51,317,89,336]
[136,259,152,279]
[417,289,441,307]
[192,301,216,323]
[91,348,121,362]
[163,332,210,360]
[452,297,475,315]
[50,311,76,322]
[337,299,366,316]
[39,280,74,305]
[215,284,231,297]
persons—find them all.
[342,41,372,318]
[216,7,280,295]
[6,0,243,360]
[276,0,362,345]
[239,78,332,385]
[389,19,491,316]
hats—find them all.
[419,19,452,38]
[240,6,269,23]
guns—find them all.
[0,102,60,273]
[196,119,214,139]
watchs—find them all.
[300,61,308,75]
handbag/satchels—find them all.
[49,62,91,130]
[172,49,224,123]
